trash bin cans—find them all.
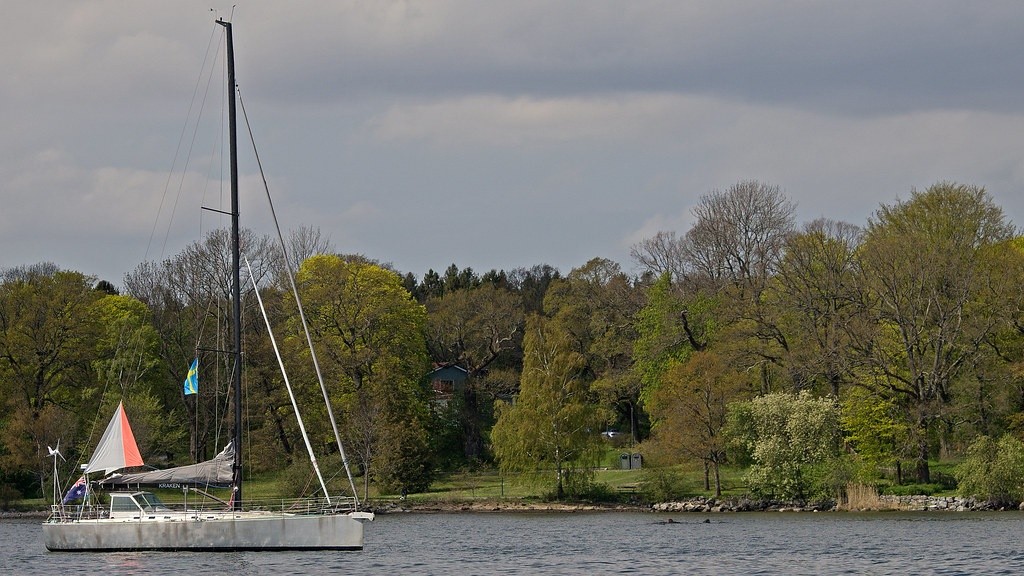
[631,453,643,469]
[619,452,631,470]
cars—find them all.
[599,431,619,444]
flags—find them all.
[63,476,87,504]
[184,358,198,396]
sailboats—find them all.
[38,11,376,552]
